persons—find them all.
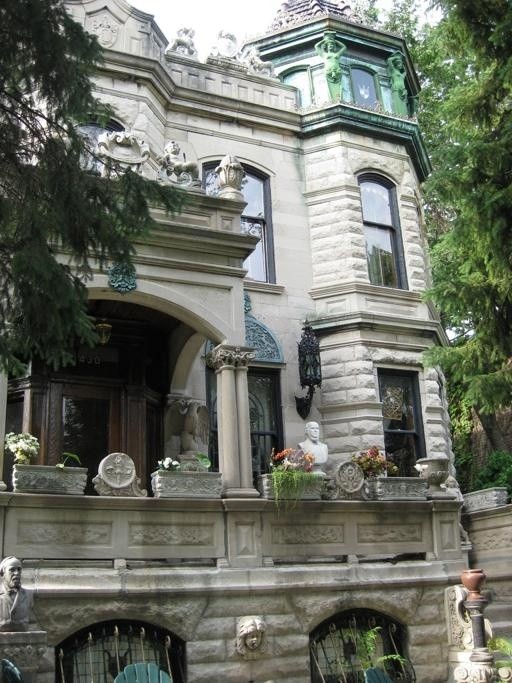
[0,553,33,631]
[299,421,329,463]
[387,54,408,117]
[314,37,347,102]
[164,139,202,179]
[236,614,268,659]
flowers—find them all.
[155,456,181,470]
[351,447,399,478]
[270,448,316,472]
[4,432,41,466]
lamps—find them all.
[94,315,113,344]
[292,314,323,421]
